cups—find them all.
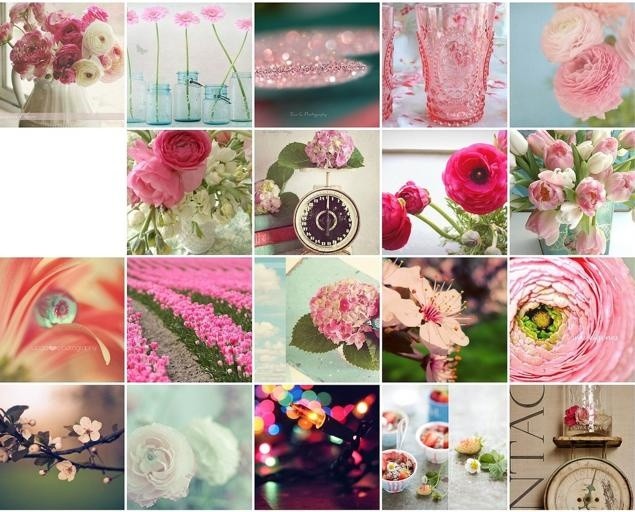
[415,3,497,126]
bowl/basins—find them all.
[415,420,448,465]
[381,449,418,495]
[381,406,410,448]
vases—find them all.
[19,78,98,127]
[538,201,613,255]
[125,72,251,126]
[175,213,217,254]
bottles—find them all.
[230,71,253,124]
[127,68,147,125]
[202,83,231,125]
[174,69,202,122]
[148,82,172,125]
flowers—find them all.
[253,161,294,216]
[125,3,252,74]
[381,129,508,254]
[127,130,253,257]
[0,2,123,87]
[278,129,365,169]
[511,130,635,255]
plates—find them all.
[255,58,378,96]
[254,30,379,59]
